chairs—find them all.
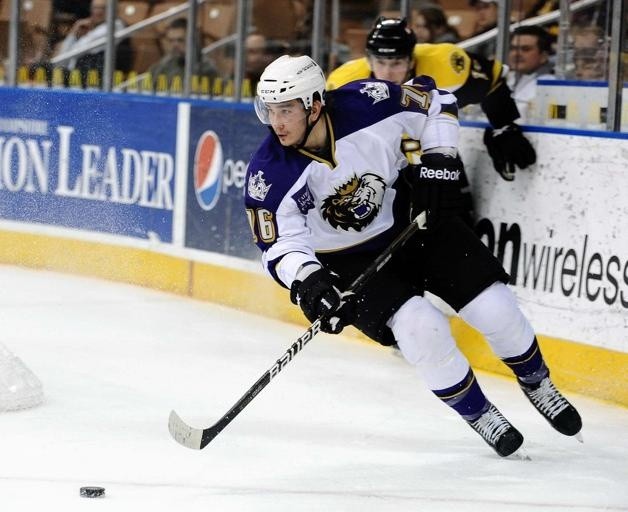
[0,0,541,79]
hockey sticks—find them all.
[167,205,427,450]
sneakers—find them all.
[431,367,524,458]
[503,337,583,437]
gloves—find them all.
[413,153,470,220]
[290,260,361,335]
[485,125,538,183]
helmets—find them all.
[252,53,328,127]
[365,14,420,59]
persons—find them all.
[469,0,499,36]
[326,16,537,229]
[224,33,273,93]
[242,52,585,461]
[411,5,461,44]
[151,18,218,86]
[506,25,558,127]
[51,0,133,81]
[526,0,559,42]
